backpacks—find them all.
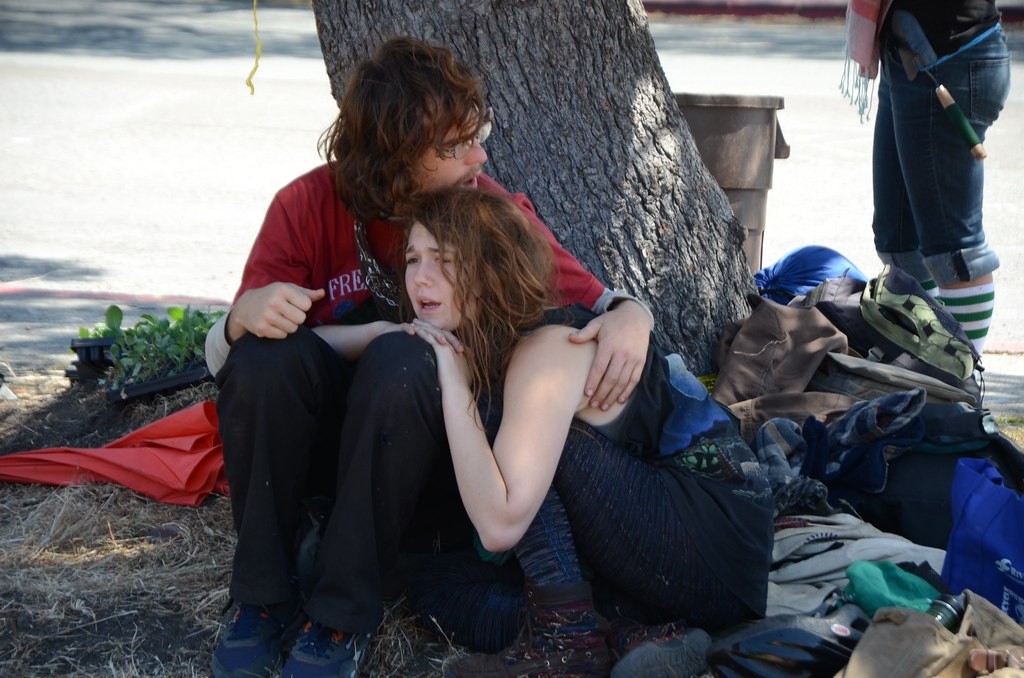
[853,402,1024,550]
[805,263,986,409]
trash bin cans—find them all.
[673,92,792,276]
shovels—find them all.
[891,10,987,161]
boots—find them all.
[608,616,712,678]
[442,580,612,678]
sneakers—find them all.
[280,617,371,678]
[212,599,300,678]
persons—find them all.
[205,34,657,678]
[838,0,1010,360]
[312,187,776,678]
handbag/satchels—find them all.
[833,589,1024,678]
[941,458,1024,629]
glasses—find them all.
[431,119,492,159]
